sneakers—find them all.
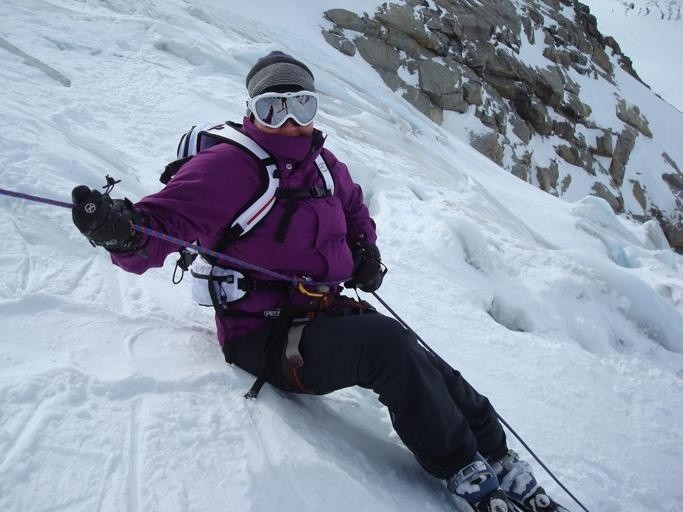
[447,451,557,512]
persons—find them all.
[71,50,567,512]
[281,97,287,109]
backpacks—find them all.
[176,121,335,306]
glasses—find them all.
[245,91,319,130]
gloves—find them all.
[71,186,142,254]
[344,240,383,292]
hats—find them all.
[246,51,313,99]
